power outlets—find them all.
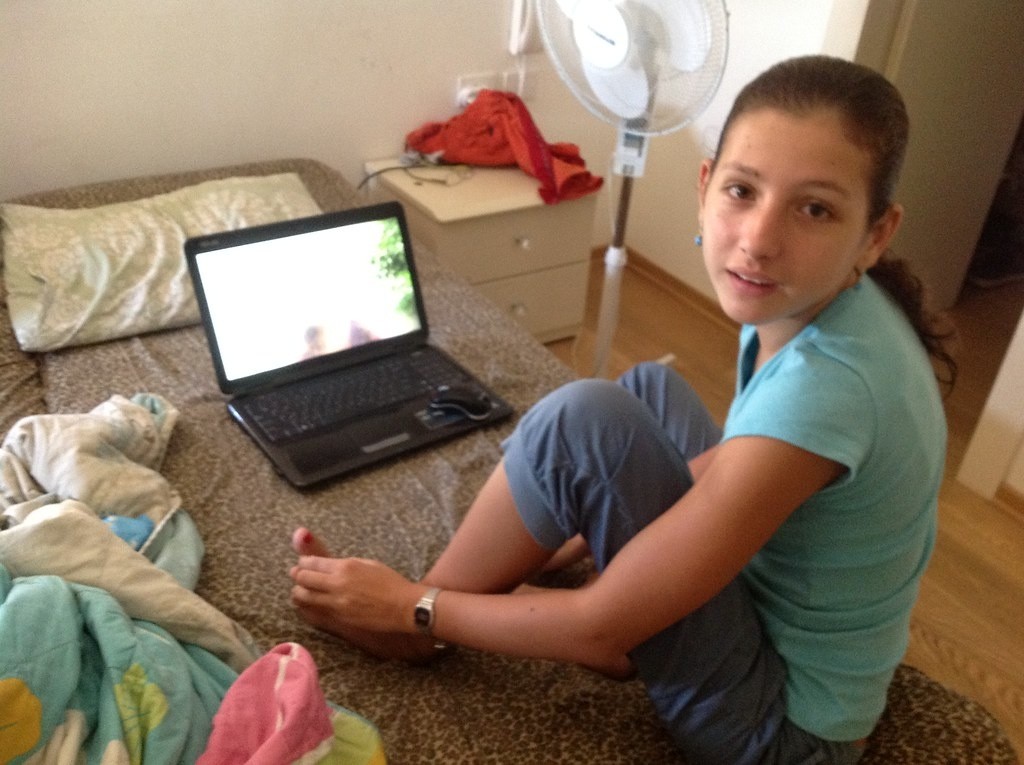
[456,71,501,93]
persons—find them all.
[287,55,946,765]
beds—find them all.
[1,158,1014,764]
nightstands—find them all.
[365,159,598,345]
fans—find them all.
[535,0,730,380]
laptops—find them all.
[184,201,516,495]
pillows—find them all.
[0,172,324,354]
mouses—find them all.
[427,382,493,423]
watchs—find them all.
[414,586,447,650]
